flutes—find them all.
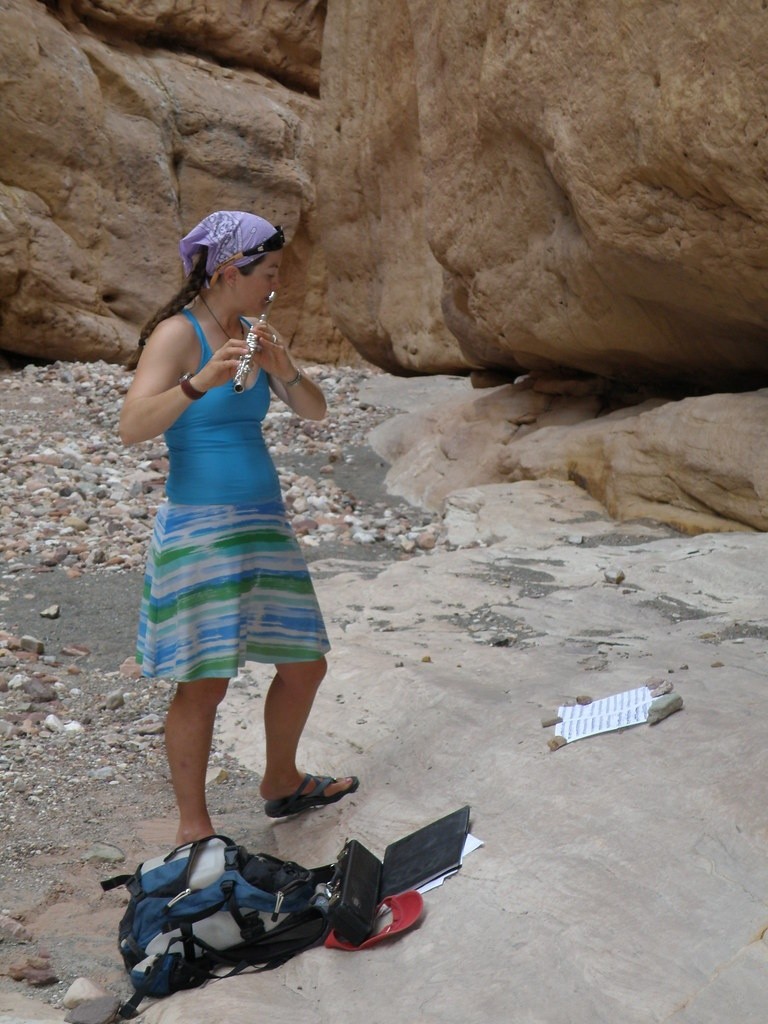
[232,289,277,395]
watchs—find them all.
[178,372,207,400]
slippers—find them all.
[264,772,359,818]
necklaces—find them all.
[198,292,244,339]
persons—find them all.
[120,211,359,847]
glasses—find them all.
[209,224,285,289]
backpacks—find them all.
[99,834,337,1020]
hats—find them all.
[324,890,424,952]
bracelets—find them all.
[287,368,302,386]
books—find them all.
[377,804,471,904]
